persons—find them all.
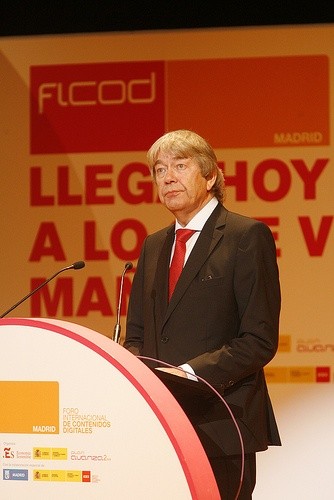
[120,130,285,500]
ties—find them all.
[169,228,216,339]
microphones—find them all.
[112,260,133,344]
[0,260,85,319]
